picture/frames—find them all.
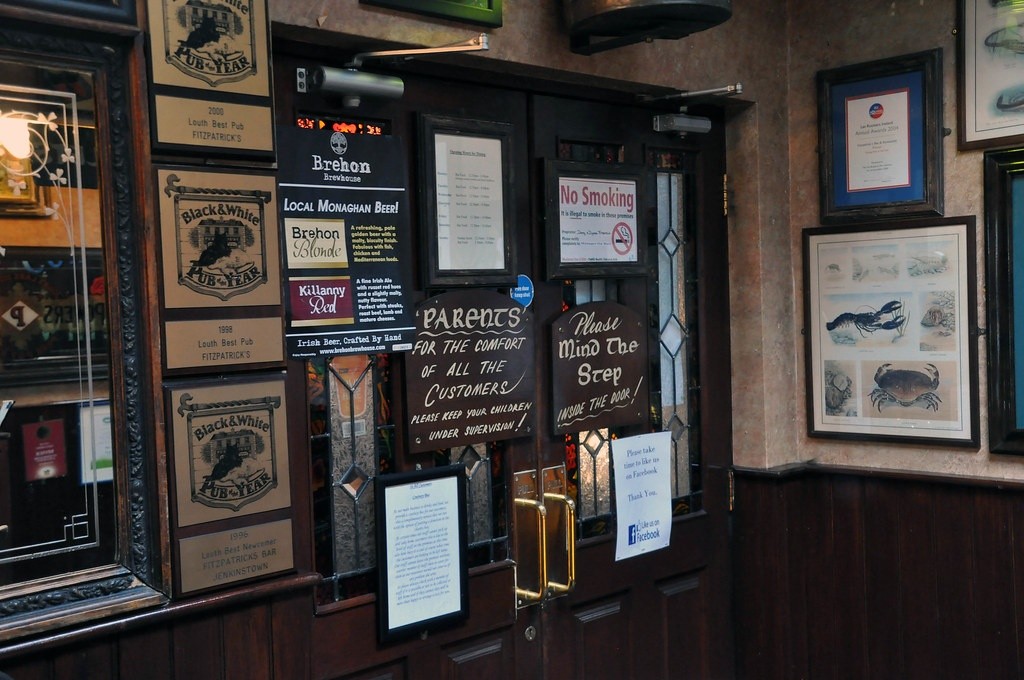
[800,0,1024,458]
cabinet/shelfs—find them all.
[0,52,134,600]
